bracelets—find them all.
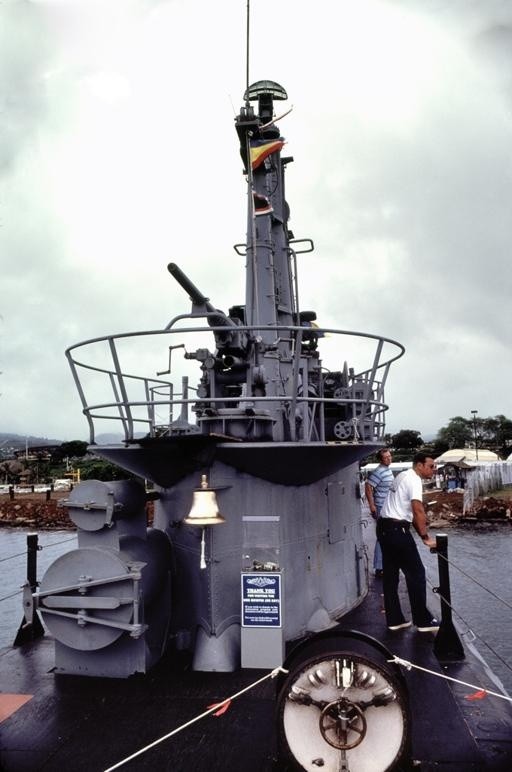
[419,533,429,541]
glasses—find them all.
[427,464,434,470]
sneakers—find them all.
[388,620,412,630]
[417,618,442,632]
[376,569,383,576]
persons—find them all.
[364,448,395,578]
[377,448,442,633]
